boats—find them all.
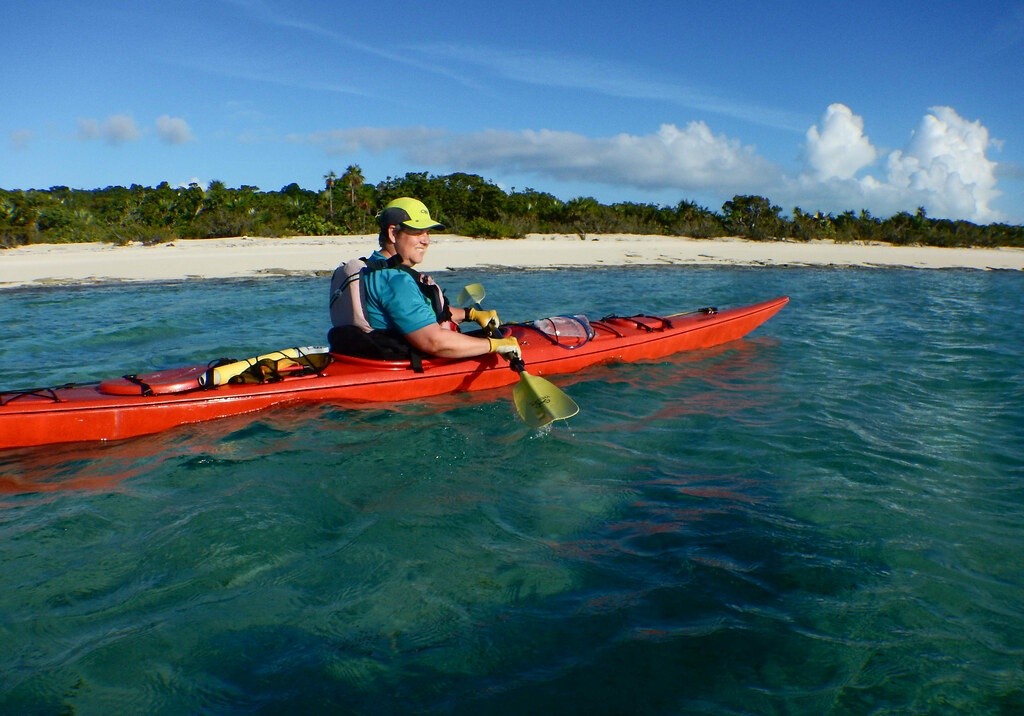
[1,295,791,453]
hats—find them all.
[375,197,446,232]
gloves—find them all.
[465,306,500,329]
[487,335,522,355]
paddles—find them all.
[456,280,580,428]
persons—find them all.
[353,197,523,361]
